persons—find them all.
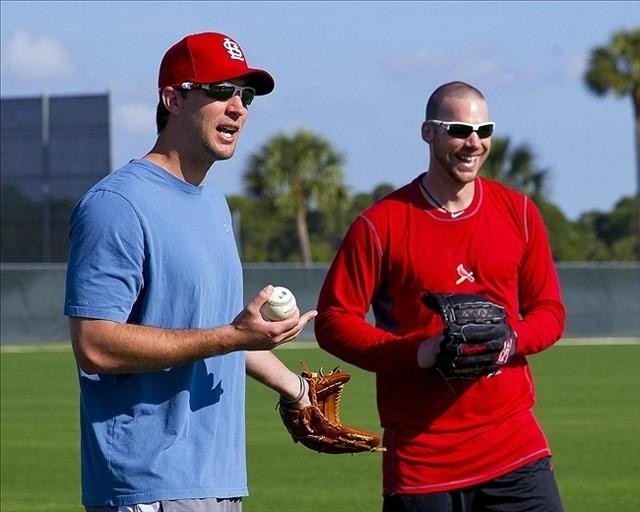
[313,80,569,512]
[62,32,386,512]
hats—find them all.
[157,31,275,96]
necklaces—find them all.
[418,179,452,214]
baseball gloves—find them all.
[421,289,513,386]
[279,364,381,454]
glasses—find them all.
[180,82,256,107]
[426,118,496,139]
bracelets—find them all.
[280,373,306,405]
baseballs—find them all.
[259,286,297,323]
